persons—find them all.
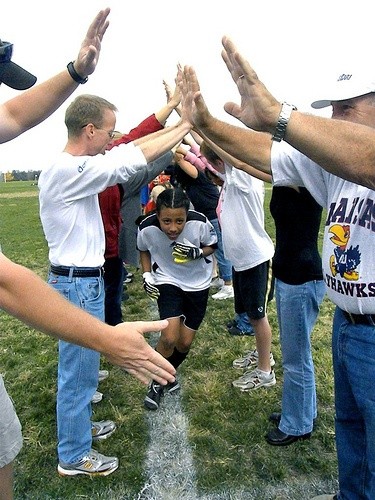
[137,176,218,411]
[163,64,326,446]
[38,79,277,476]
[180,35,375,500]
[0,5,177,500]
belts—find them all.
[342,310,375,326]
[49,266,106,277]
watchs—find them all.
[272,101,297,142]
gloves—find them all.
[142,272,161,301]
[170,239,203,263]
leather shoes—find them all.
[271,410,316,427]
[265,427,310,445]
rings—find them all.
[239,75,244,78]
[98,34,101,36]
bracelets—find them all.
[68,60,88,83]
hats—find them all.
[310,68,375,109]
[0,41,38,91]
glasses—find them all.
[80,123,116,140]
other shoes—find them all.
[228,326,255,336]
[91,391,104,403]
[227,319,239,327]
[98,369,109,381]
[120,272,134,301]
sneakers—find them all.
[57,448,120,477]
[144,380,163,410]
[232,349,275,369]
[210,277,224,288]
[211,285,235,299]
[166,378,181,395]
[90,419,117,440]
[232,366,276,392]
